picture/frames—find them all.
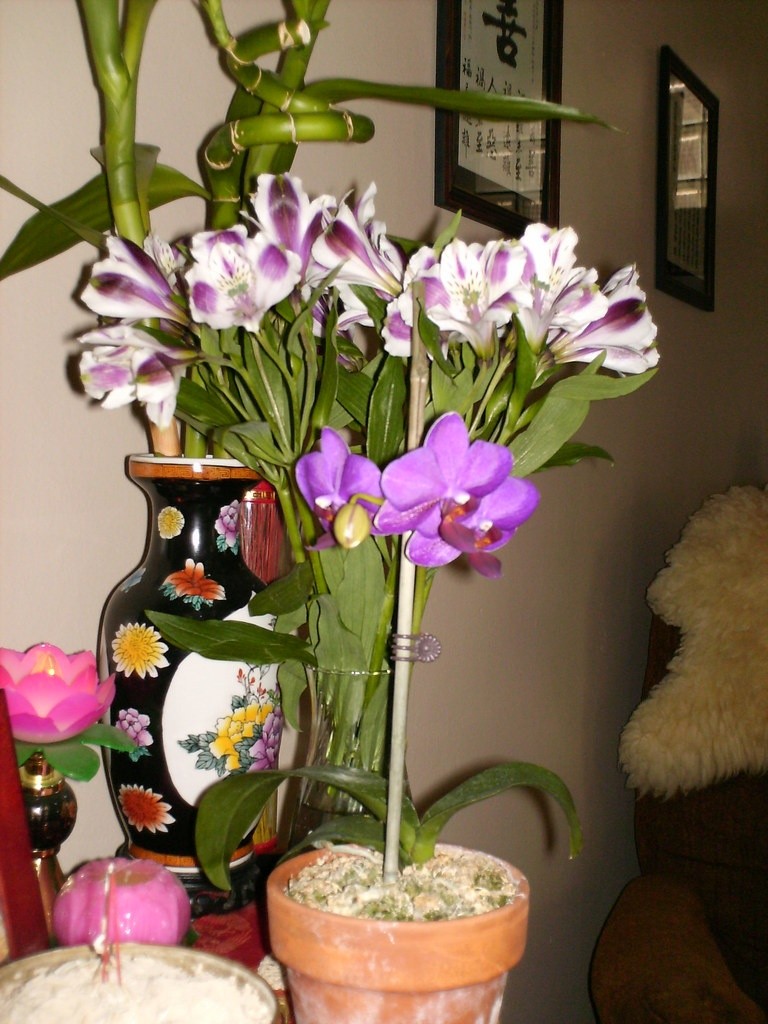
[434,0,564,242]
[654,45,720,313]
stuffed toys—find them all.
[620,484,768,804]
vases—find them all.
[98,454,287,880]
[16,746,77,938]
[258,839,536,1024]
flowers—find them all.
[0,632,140,786]
[74,167,677,890]
